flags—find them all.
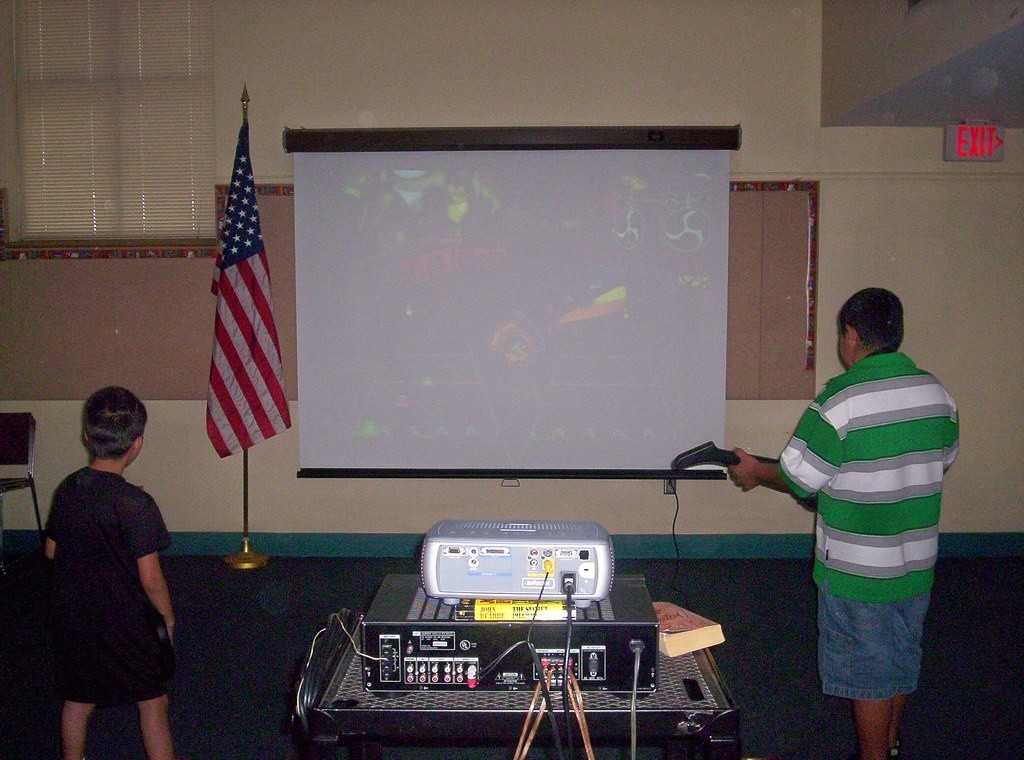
[204,120,292,460]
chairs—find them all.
[0,411,46,548]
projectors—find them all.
[420,520,615,603]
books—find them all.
[652,601,726,658]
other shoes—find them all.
[885,739,899,760]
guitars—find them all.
[670,439,817,512]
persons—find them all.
[726,287,958,760]
[43,386,180,760]
